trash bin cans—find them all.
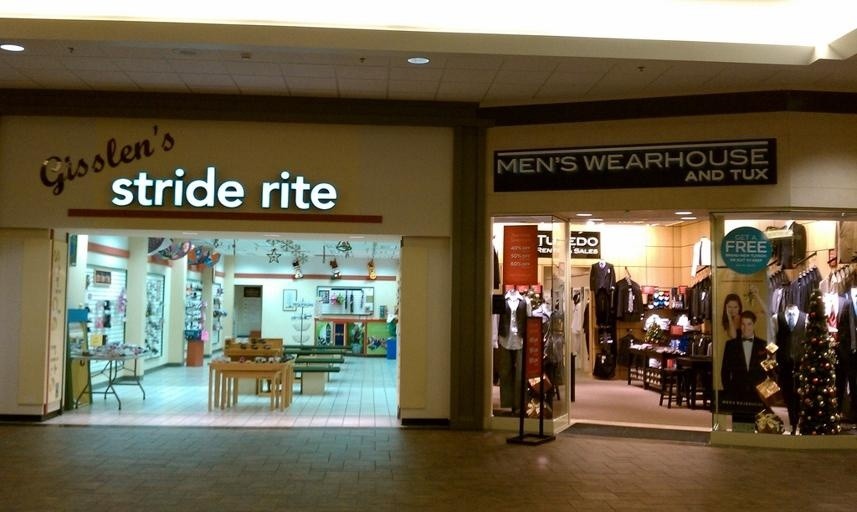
[386,338,396,359]
[186,339,204,367]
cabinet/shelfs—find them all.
[588,287,689,380]
[314,319,388,357]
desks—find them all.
[627,346,686,395]
[677,357,713,409]
[71,350,152,409]
[208,357,296,412]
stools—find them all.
[659,368,691,408]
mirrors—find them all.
[67,321,93,409]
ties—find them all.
[699,241,703,266]
[628,288,634,312]
[855,294,857,316]
[788,313,795,331]
[600,263,603,269]
[828,304,837,338]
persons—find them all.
[719,294,767,389]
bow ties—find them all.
[742,337,753,342]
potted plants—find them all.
[644,321,667,347]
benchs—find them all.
[283,345,353,395]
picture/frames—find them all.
[283,289,297,311]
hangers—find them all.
[617,267,641,292]
[766,252,857,284]
